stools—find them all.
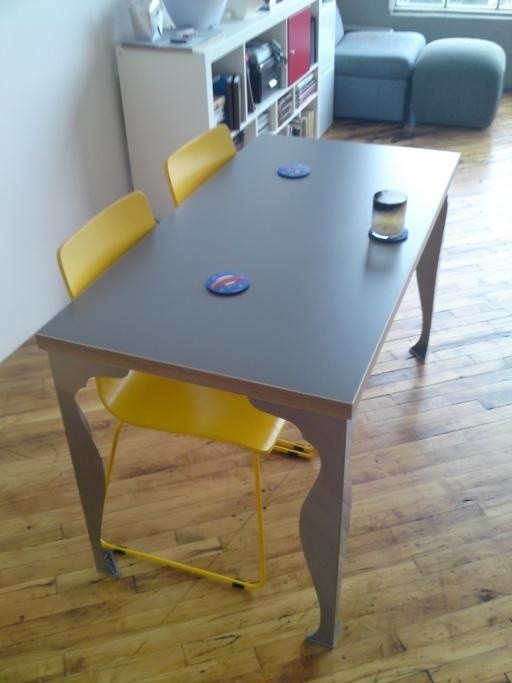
[409,36,508,131]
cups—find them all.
[370,189,408,239]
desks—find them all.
[36,132,462,651]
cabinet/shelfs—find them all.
[115,0,320,223]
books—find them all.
[210,16,322,153]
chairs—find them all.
[55,189,318,592]
[335,7,427,123]
[163,125,321,454]
